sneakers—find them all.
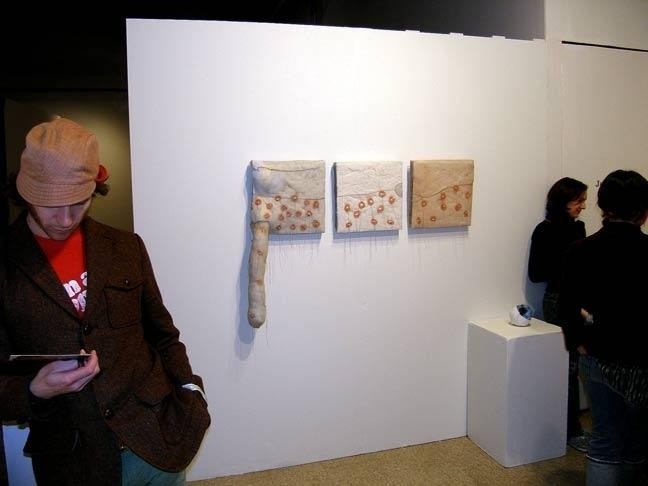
[567,436,589,453]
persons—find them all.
[560,169,647,485]
[522,178,593,454]
[0,117,212,486]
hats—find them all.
[15,117,101,208]
